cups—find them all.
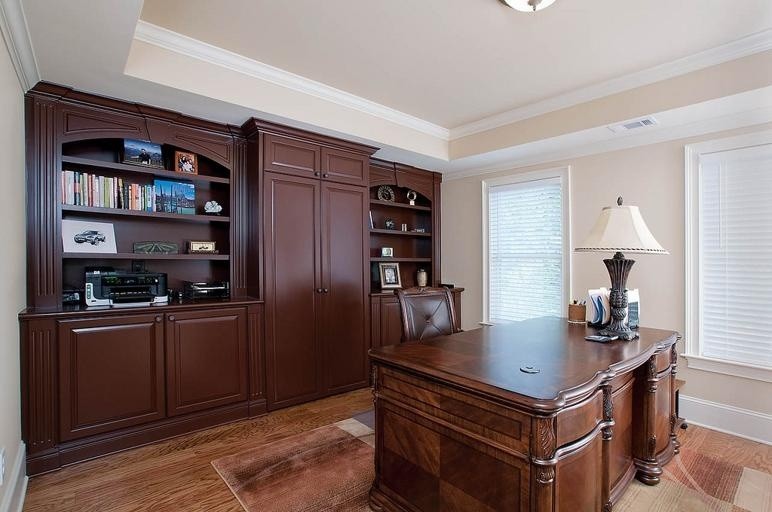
[568,304,586,322]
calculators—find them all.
[584,334,619,343]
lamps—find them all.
[573,197,669,341]
[500,0,556,12]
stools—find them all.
[675,379,688,429]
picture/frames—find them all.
[378,262,404,290]
[175,150,198,175]
[120,138,168,170]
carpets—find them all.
[211,410,772,511]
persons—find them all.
[178,156,186,168]
[183,161,194,172]
[138,148,152,166]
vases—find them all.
[417,269,428,287]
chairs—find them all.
[398,287,464,342]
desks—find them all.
[367,316,681,512]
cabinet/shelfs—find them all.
[240,117,381,412]
[18,81,265,476]
[369,157,464,386]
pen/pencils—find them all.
[570,300,586,305]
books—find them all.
[587,287,641,329]
[369,211,374,229]
[153,178,180,213]
[171,182,197,216]
[58,170,157,213]
